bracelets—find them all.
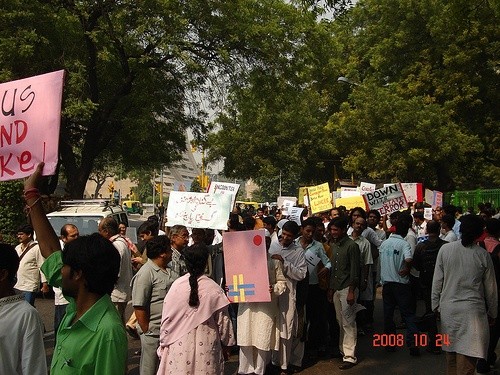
[24,188,41,216]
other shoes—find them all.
[409,347,421,355]
[358,329,364,336]
[126,326,135,337]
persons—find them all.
[24,161,128,375]
[52,224,79,333]
[98,203,500,375]
[13,226,50,306]
[0,243,48,375]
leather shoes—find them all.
[339,360,357,369]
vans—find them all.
[32,199,166,255]
